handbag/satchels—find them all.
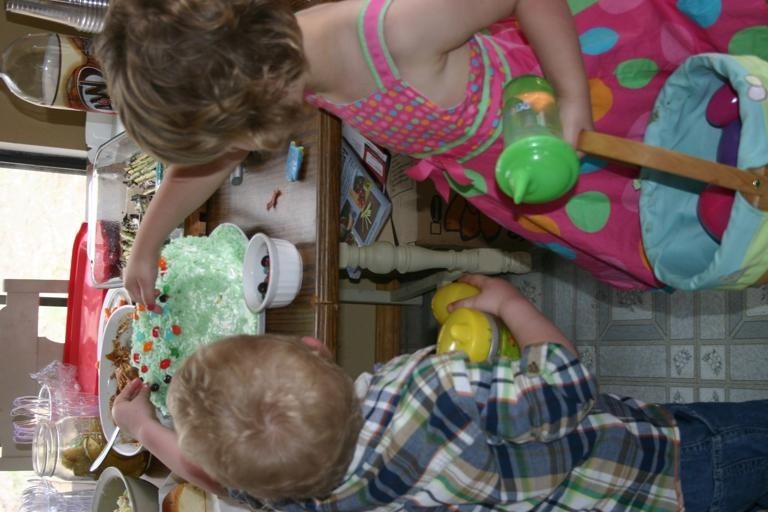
[572,45,768,292]
